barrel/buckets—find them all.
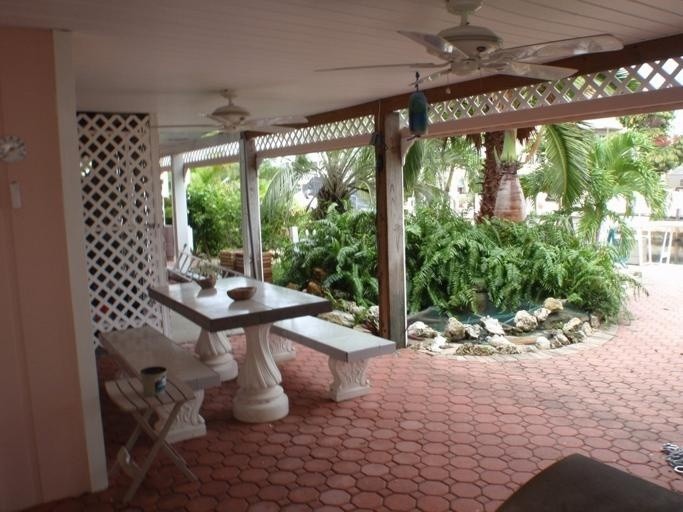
[140,366,167,396]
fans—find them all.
[150,89,309,140]
[313,0,624,85]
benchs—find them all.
[270,315,396,402]
[99,326,220,443]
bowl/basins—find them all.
[227,285,256,301]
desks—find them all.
[105,377,196,505]
[149,277,331,424]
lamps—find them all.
[410,72,429,136]
